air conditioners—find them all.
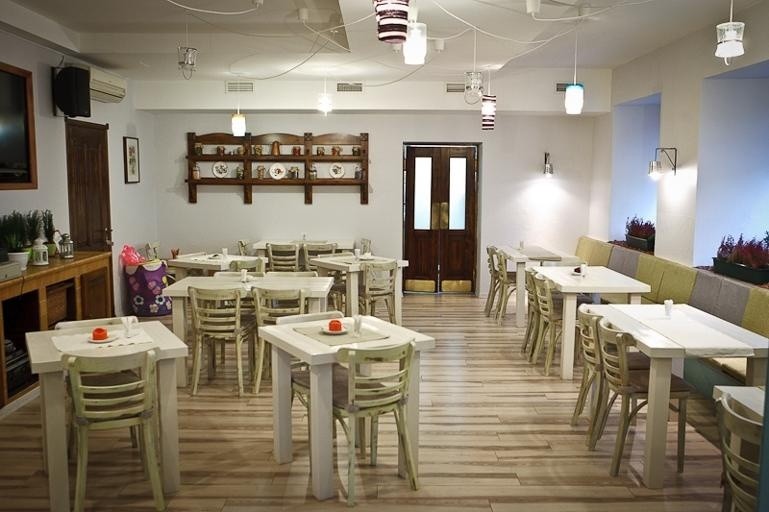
[64,62,127,104]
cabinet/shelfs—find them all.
[0,251,112,420]
[185,132,368,204]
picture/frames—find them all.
[123,137,140,183]
[0,63,38,189]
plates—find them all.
[212,161,229,179]
[328,162,345,179]
[359,258,375,261]
[571,266,590,275]
[87,332,119,343]
[321,324,351,335]
[269,163,286,180]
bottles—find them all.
[240,269,249,283]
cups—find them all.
[354,248,361,259]
[665,303,672,316]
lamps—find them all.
[544,152,553,174]
[481,69,496,131]
[179,21,196,81]
[466,30,480,106]
[376,0,408,43]
[714,0,745,66]
[409,1,427,65]
[564,30,584,115]
[649,148,677,176]
[231,90,246,137]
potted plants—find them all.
[625,216,655,251]
[0,209,55,270]
